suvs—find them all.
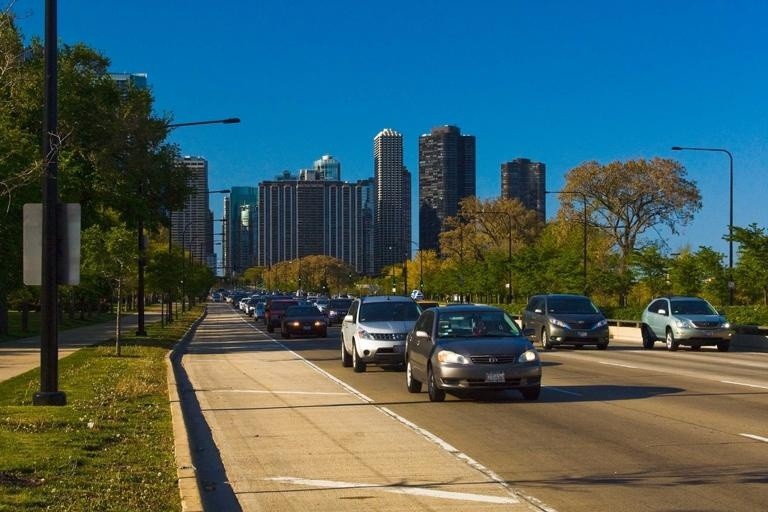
[339,294,425,374]
[640,295,740,353]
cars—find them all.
[213,288,486,339]
[402,304,542,402]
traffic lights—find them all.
[420,281,424,288]
[392,281,396,286]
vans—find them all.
[520,293,609,350]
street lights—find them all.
[275,240,464,304]
[540,190,588,294]
[475,210,512,303]
[167,189,230,324]
[133,116,241,336]
[672,145,734,305]
